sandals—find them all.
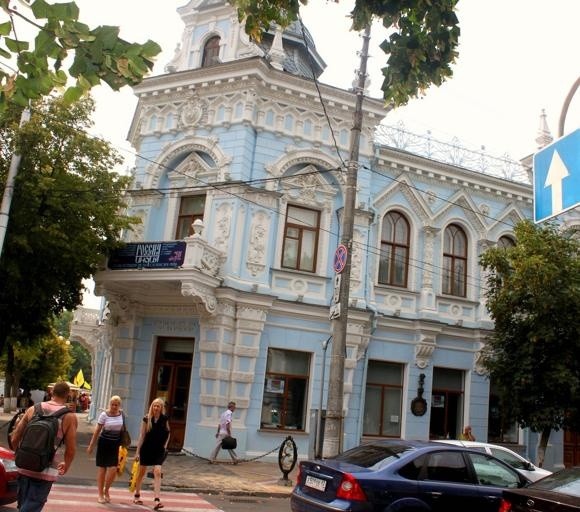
[132,495,144,505]
[151,497,165,511]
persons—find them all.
[132,396,172,511]
[208,401,243,465]
[64,388,91,412]
[86,394,127,504]
[41,384,55,402]
[458,425,476,443]
[7,397,34,454]
[9,382,78,512]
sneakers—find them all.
[207,457,242,465]
[96,494,112,504]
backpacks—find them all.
[13,400,74,473]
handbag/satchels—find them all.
[119,410,131,447]
[221,437,237,449]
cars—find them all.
[291,439,580,512]
[0,446,17,506]
